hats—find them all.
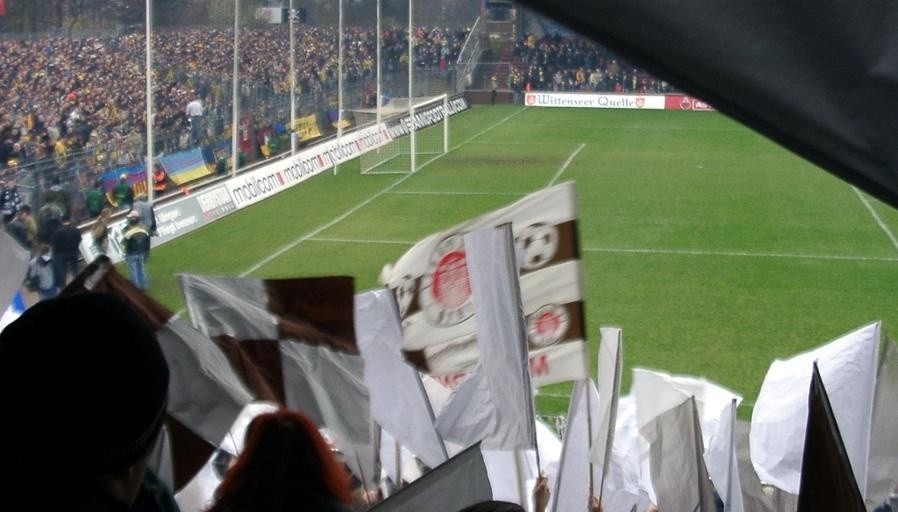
[1,292,171,475]
[126,210,139,219]
[119,173,129,180]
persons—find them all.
[0,106,345,176]
[0,0,673,105]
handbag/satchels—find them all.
[26,259,42,294]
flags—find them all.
[0,176,898,511]
